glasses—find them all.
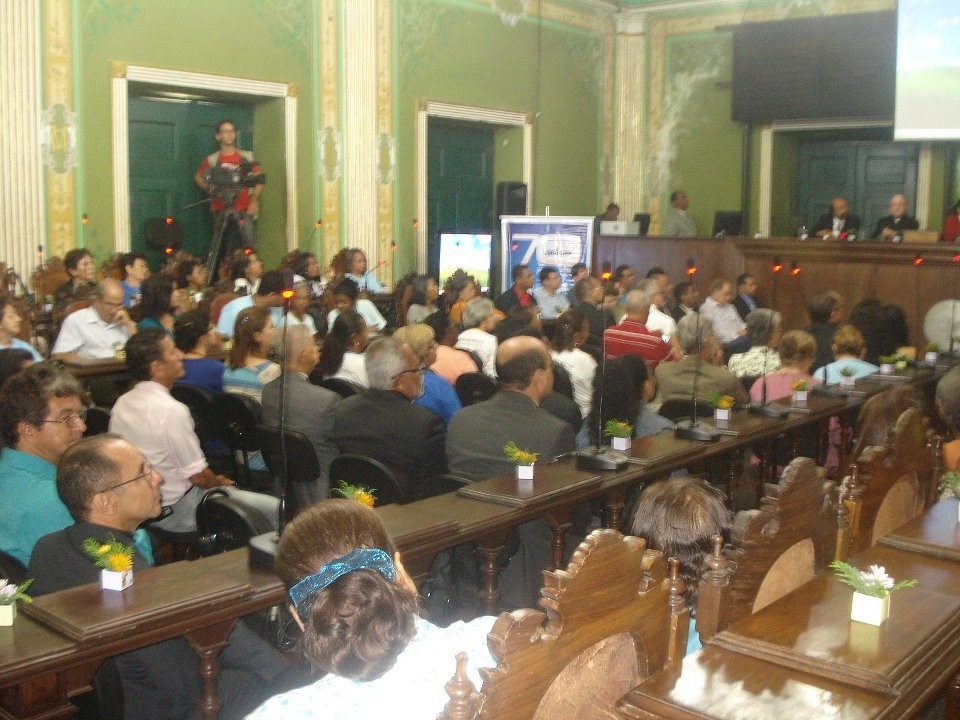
[193,269,204,274]
[207,324,217,332]
[260,320,277,335]
[427,339,439,350]
[656,290,663,295]
[833,303,849,310]
[135,263,146,269]
[99,299,124,310]
[29,406,87,428]
[391,362,426,379]
[100,457,153,494]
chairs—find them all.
[173,378,231,475]
[435,389,942,717]
[327,448,404,509]
[247,420,327,513]
[213,389,281,503]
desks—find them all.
[620,487,959,719]
[0,357,918,720]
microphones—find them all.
[750,256,789,417]
[365,261,384,296]
[941,256,960,362]
[577,261,629,470]
[36,246,52,323]
[247,269,294,569]
[165,217,175,244]
[790,265,848,399]
[160,247,173,287]
[906,258,933,369]
[305,219,320,289]
[671,258,723,441]
[81,214,88,285]
[243,248,252,268]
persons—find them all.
[871,193,919,241]
[238,502,507,720]
[938,197,960,242]
[622,475,740,653]
[661,189,696,237]
[2,229,960,601]
[194,120,264,286]
[809,196,861,242]
[593,202,620,236]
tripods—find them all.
[206,209,253,282]
